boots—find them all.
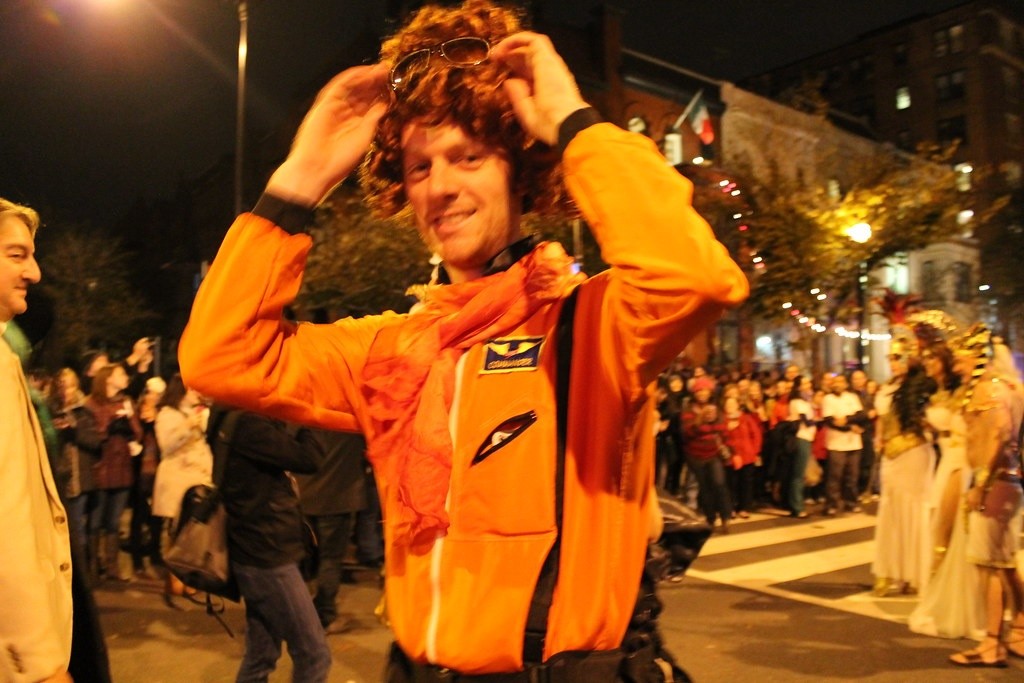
[87,533,99,588]
[107,533,120,581]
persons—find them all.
[0,200,1024,683]
[178,3,751,683]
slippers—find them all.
[949,647,1007,668]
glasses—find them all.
[390,37,491,86]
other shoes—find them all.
[825,506,835,515]
[850,506,860,512]
[328,612,347,633]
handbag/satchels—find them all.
[161,483,240,603]
